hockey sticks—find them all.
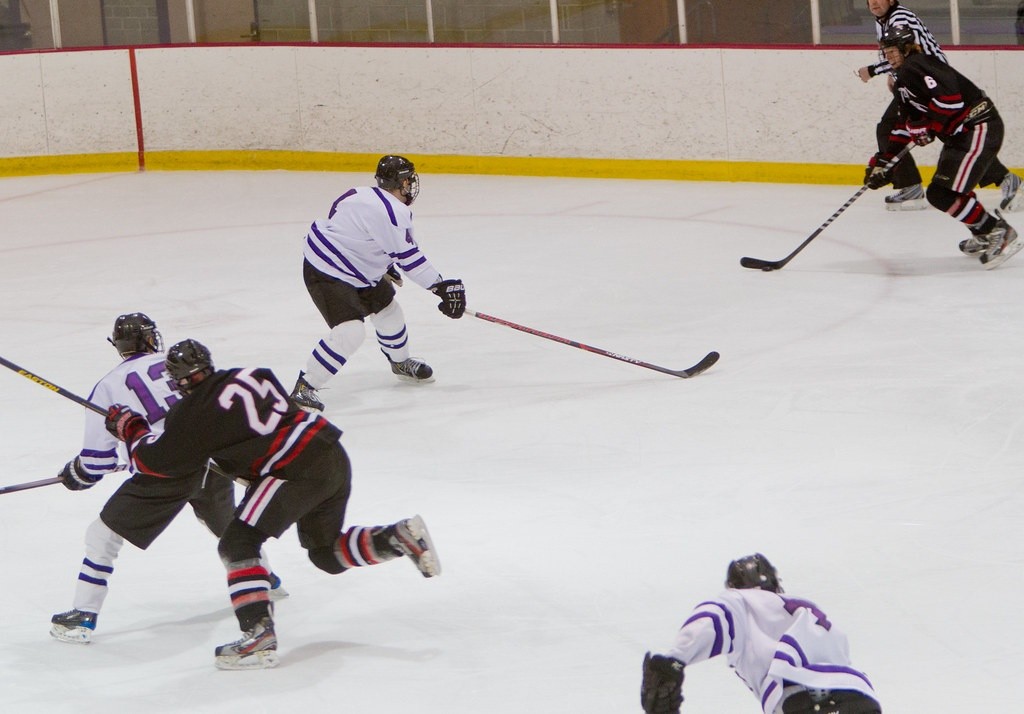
[438,301,721,379]
[738,140,917,269]
[0,476,63,495]
[0,357,250,487]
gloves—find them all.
[386,265,404,288]
[906,118,929,147]
[104,404,152,445]
[864,152,899,191]
[57,455,104,491]
[434,279,466,319]
[640,651,686,714]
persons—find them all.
[290,155,466,413]
[105,338,442,669]
[857,0,1024,270]
[642,553,883,714]
[50,312,291,644]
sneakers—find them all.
[386,515,441,578]
[884,183,930,212]
[50,610,97,645]
[214,616,280,671]
[265,572,290,601]
[383,352,436,383]
[959,232,999,257]
[289,371,325,413]
[1001,173,1023,214]
[979,219,1024,271]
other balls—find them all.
[762,267,773,272]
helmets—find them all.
[376,155,419,206]
[880,29,915,75]
[113,312,163,356]
[727,553,779,592]
[165,339,212,390]
